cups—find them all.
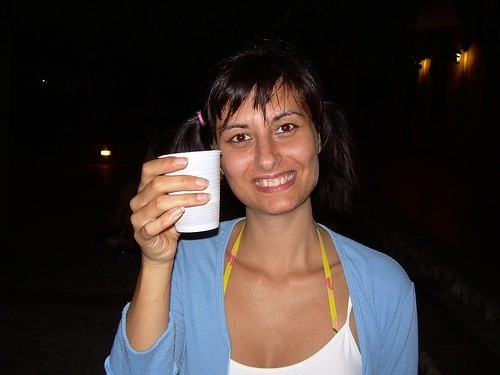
[157,149,223,233]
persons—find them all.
[103,51,419,375]
